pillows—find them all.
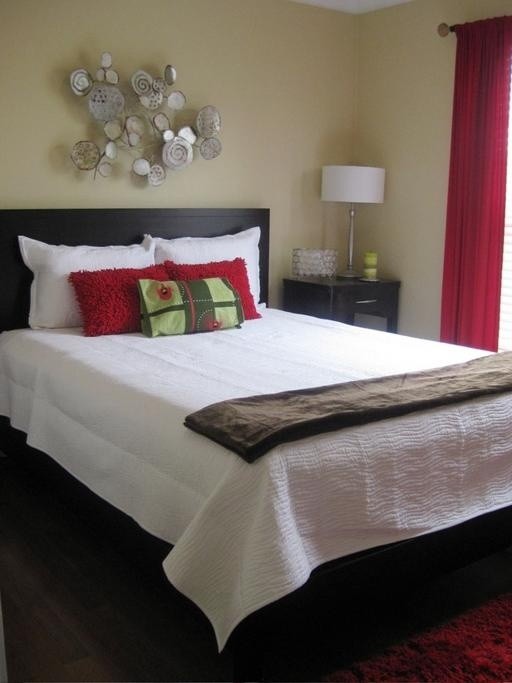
[134,273,250,338]
[169,257,262,320]
[67,262,169,338]
[14,230,155,334]
[143,222,269,316]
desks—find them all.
[281,272,401,334]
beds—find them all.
[0,205,510,681]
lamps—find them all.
[318,162,388,279]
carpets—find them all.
[332,590,511,682]
